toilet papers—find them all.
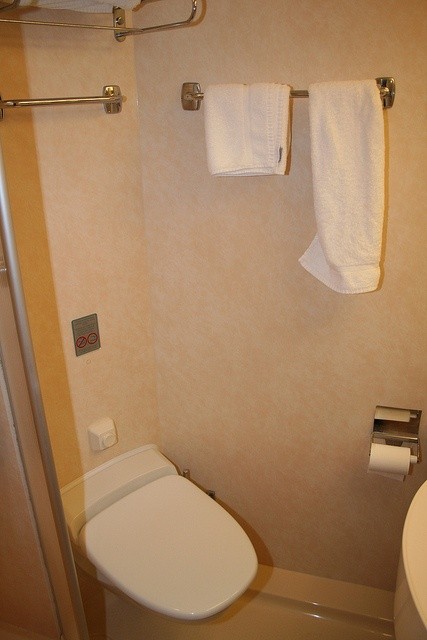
[367,443,422,483]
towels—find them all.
[16,0,140,15]
[203,81,292,178]
[298,78,387,295]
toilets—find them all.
[59,443,258,621]
